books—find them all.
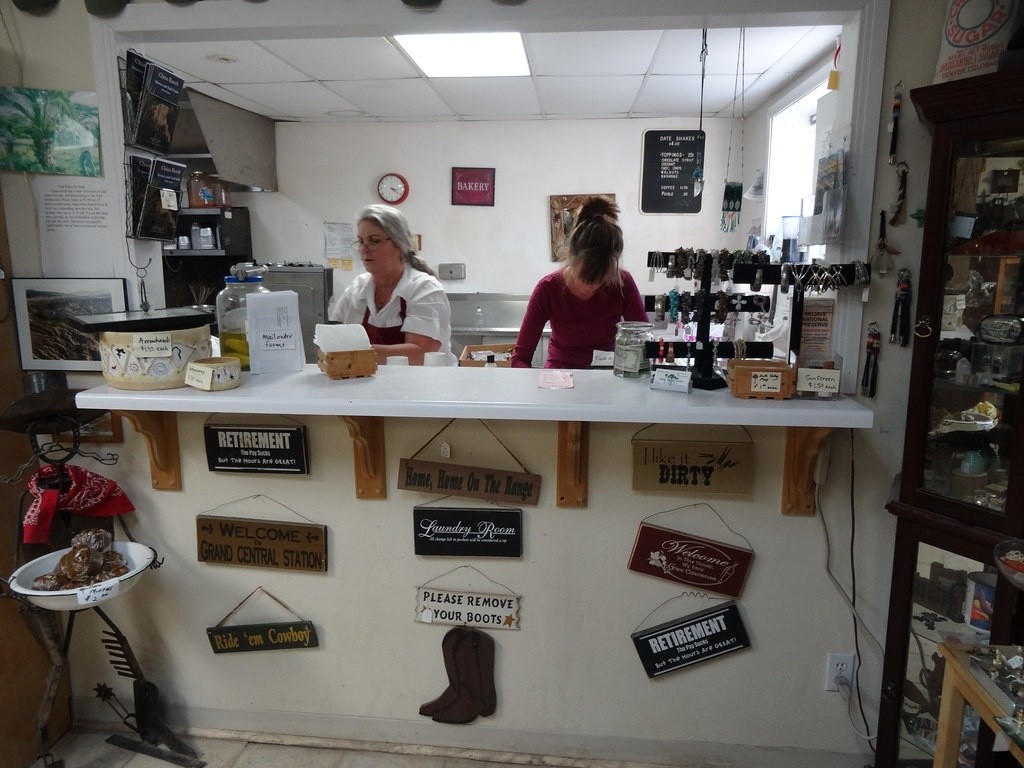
[120,48,188,243]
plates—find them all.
[992,538,1024,589]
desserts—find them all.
[31,528,130,592]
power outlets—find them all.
[825,653,855,693]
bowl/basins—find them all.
[8,541,153,611]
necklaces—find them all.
[374,271,402,308]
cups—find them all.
[193,357,242,391]
[960,451,985,475]
[780,239,799,264]
[613,322,655,379]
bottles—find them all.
[208,174,226,208]
[178,236,191,249]
[666,341,674,363]
[200,228,212,249]
[216,276,273,371]
[191,222,200,249]
[476,306,483,328]
[186,172,214,208]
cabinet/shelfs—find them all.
[160,206,252,337]
[874,66,1024,768]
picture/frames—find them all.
[995,258,1024,317]
[451,166,495,207]
[50,412,123,443]
[12,278,129,373]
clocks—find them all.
[378,173,409,205]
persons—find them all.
[4,125,18,152]
[510,195,651,370]
[327,204,459,368]
[76,124,96,177]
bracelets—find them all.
[650,245,870,374]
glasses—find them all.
[351,237,390,249]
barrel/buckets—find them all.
[782,216,801,239]
[964,571,997,636]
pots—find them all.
[98,324,212,390]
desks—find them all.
[932,641,1024,768]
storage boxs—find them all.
[791,351,842,402]
[941,295,965,332]
[787,297,833,363]
[727,358,796,400]
[318,349,378,381]
[459,344,514,368]
